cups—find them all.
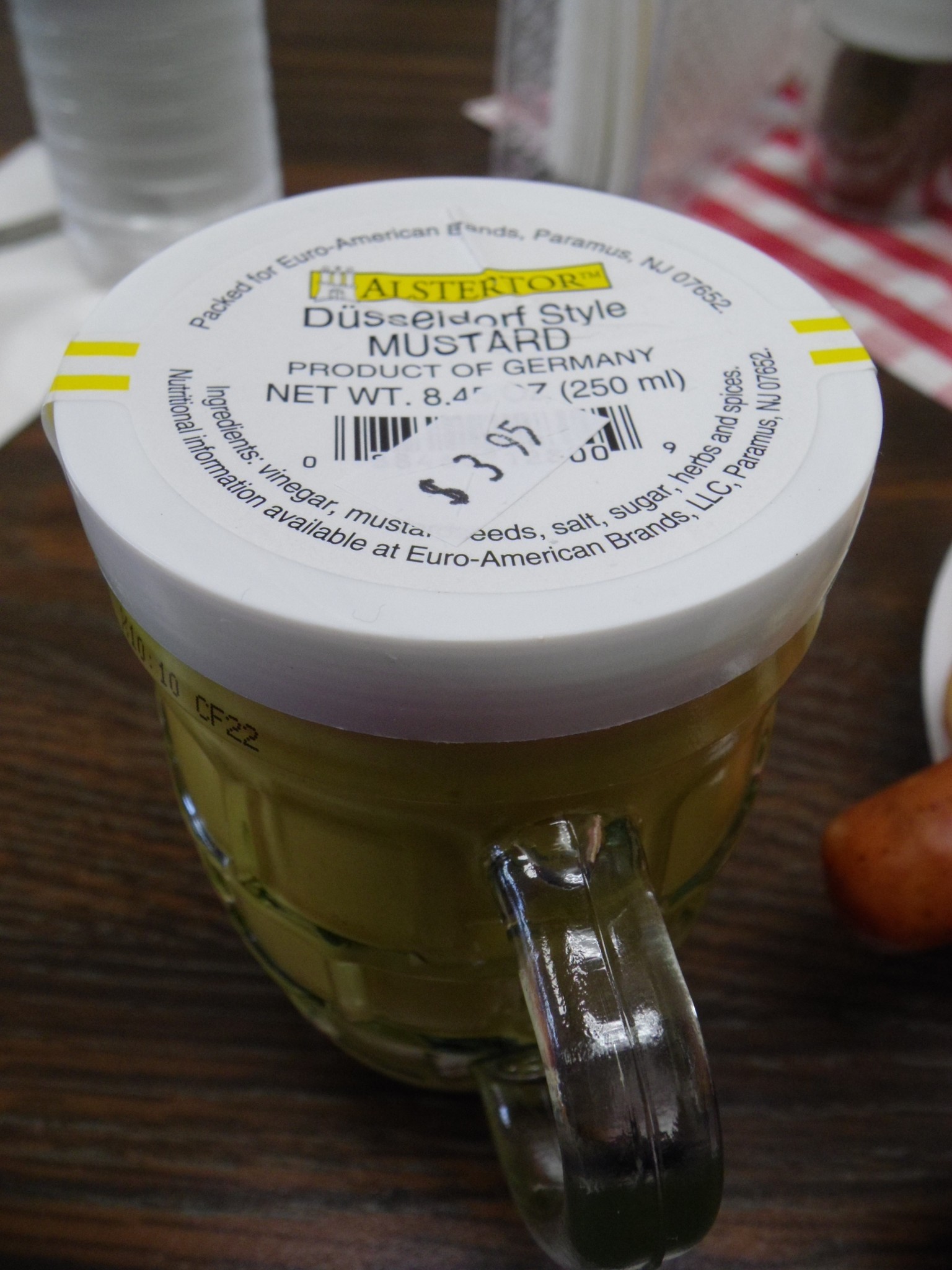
[488,1,704,205]
[9,0,285,286]
[794,0,952,220]
[39,173,882,1269]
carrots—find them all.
[821,753,952,954]
